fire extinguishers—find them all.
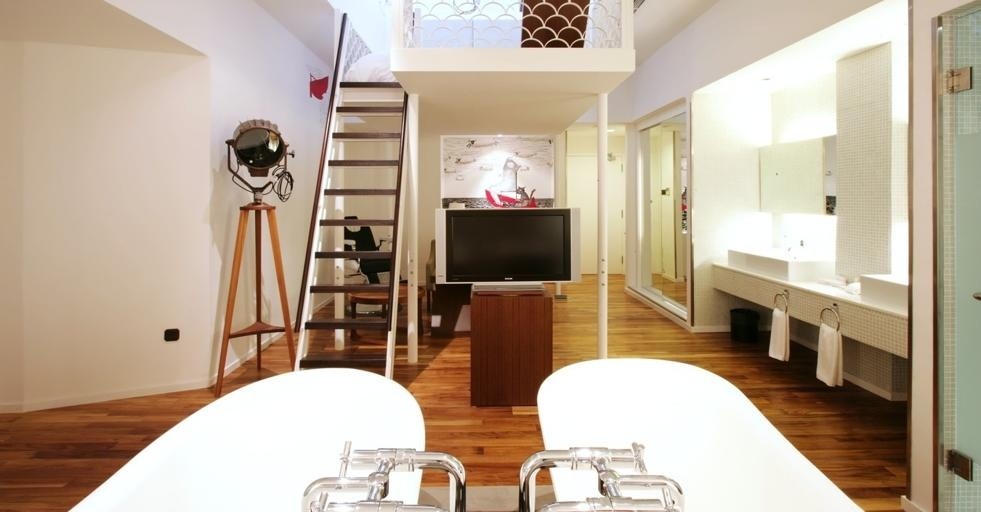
[681,186,688,212]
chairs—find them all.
[426,240,436,313]
[344,216,402,317]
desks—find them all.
[347,284,425,337]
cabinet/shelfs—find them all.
[471,296,553,406]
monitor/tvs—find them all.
[435,206,581,290]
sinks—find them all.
[856,273,910,315]
[727,247,835,283]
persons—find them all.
[344,217,391,284]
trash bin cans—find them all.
[730,308,759,342]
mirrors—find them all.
[823,135,838,215]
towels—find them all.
[816,323,844,388]
[768,307,789,362]
